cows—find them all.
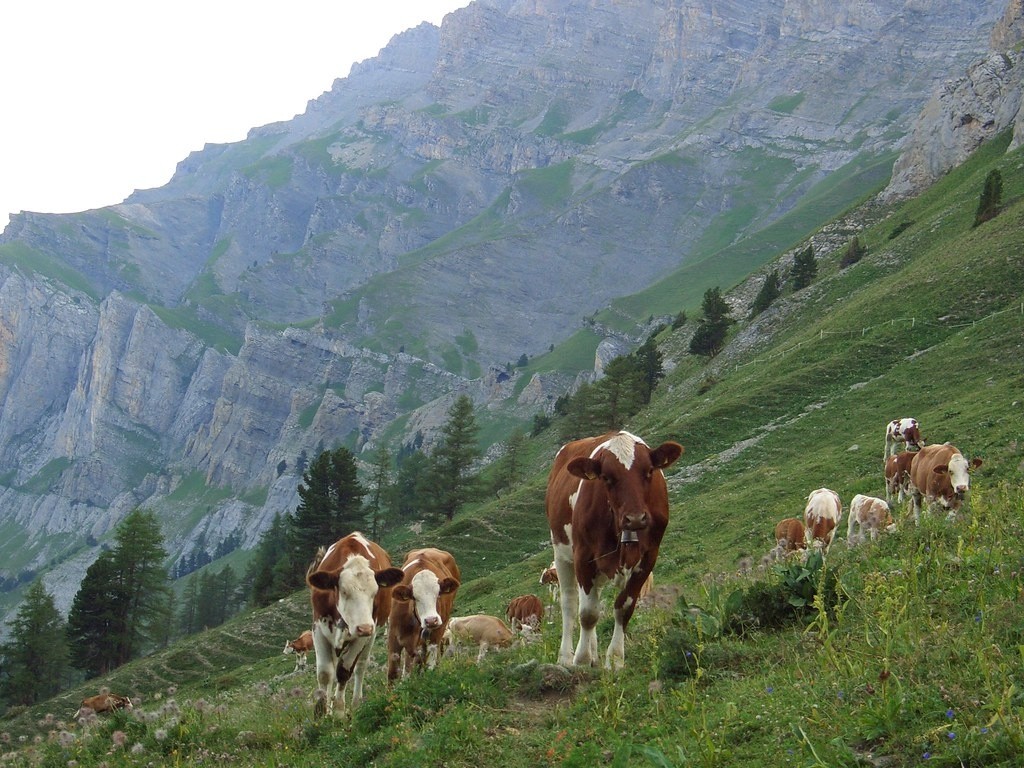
[539,427,686,674]
[304,524,462,734]
[847,493,899,551]
[71,689,134,719]
[883,416,929,463]
[442,611,516,671]
[774,517,809,560]
[281,629,315,674]
[504,594,545,648]
[803,487,844,559]
[883,440,983,529]
